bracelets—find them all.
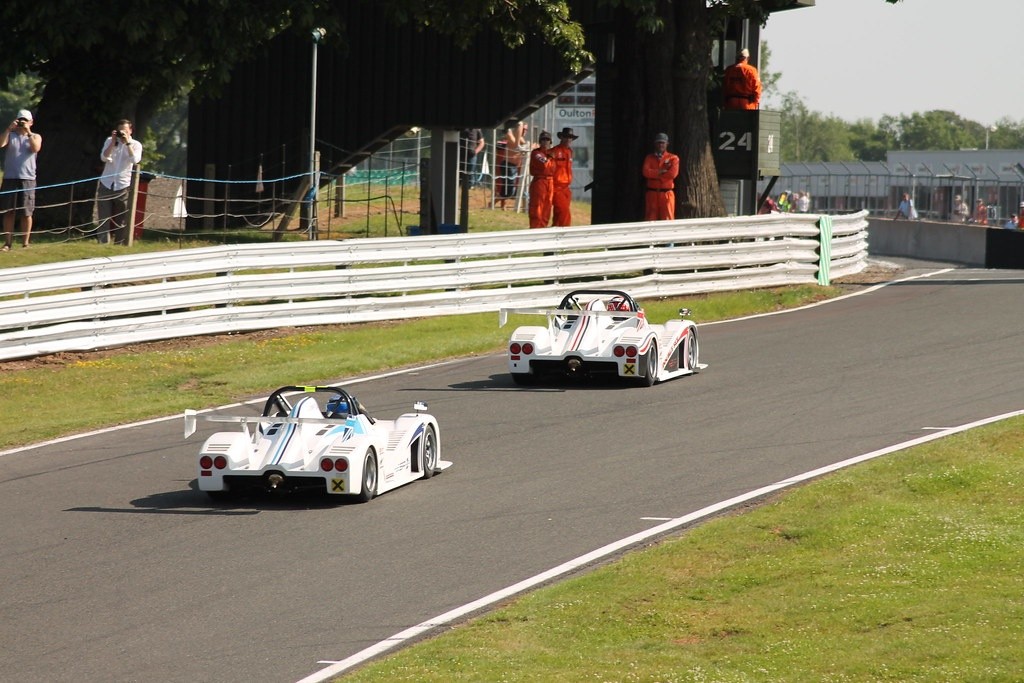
[28,135,32,137]
[126,143,130,145]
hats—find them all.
[16,109,33,121]
[537,131,553,145]
[654,133,670,144]
[556,127,580,141]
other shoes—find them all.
[0,245,13,252]
[23,245,29,249]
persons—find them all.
[893,193,917,221]
[643,133,679,220]
[0,109,42,252]
[489,122,539,210]
[971,199,988,224]
[459,129,485,186]
[775,190,810,213]
[530,132,555,228]
[98,119,142,244]
[608,296,631,310]
[553,128,580,227]
[1006,202,1024,227]
[724,48,761,109]
[950,195,968,223]
[326,394,348,413]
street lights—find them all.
[306,27,326,240]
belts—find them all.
[645,186,672,193]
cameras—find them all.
[115,131,123,137]
[17,120,24,127]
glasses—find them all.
[541,138,550,141]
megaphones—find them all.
[311,28,326,42]
